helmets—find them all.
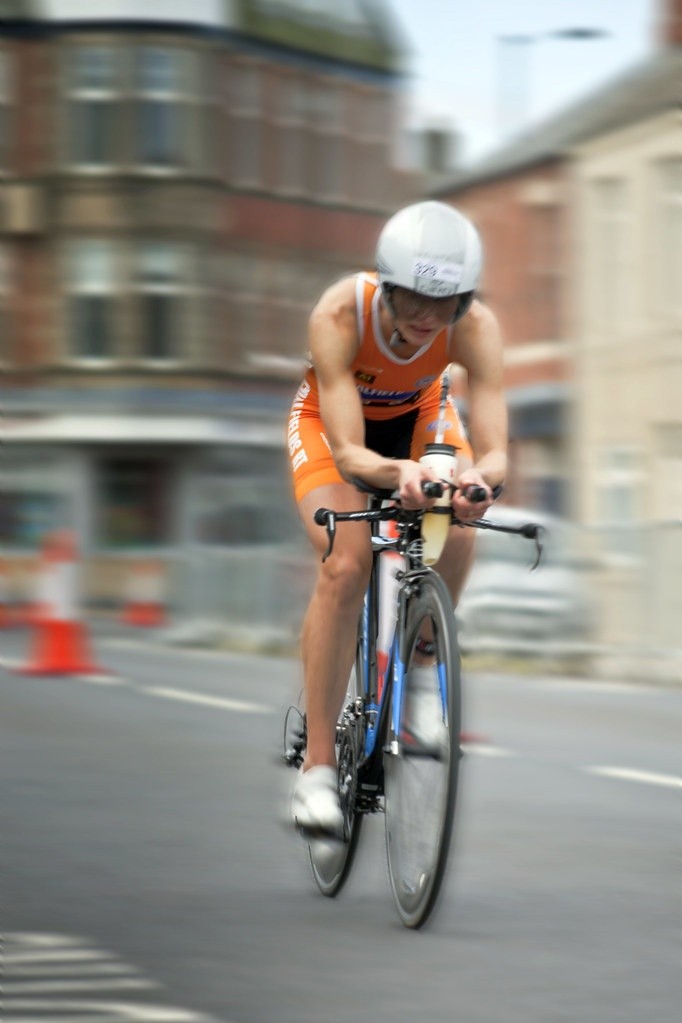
[374,199,483,324]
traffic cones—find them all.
[5,529,109,673]
[120,546,172,628]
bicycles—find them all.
[293,484,540,928]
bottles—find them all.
[415,440,458,567]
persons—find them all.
[272,199,512,831]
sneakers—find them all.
[403,665,451,753]
[290,762,345,829]
